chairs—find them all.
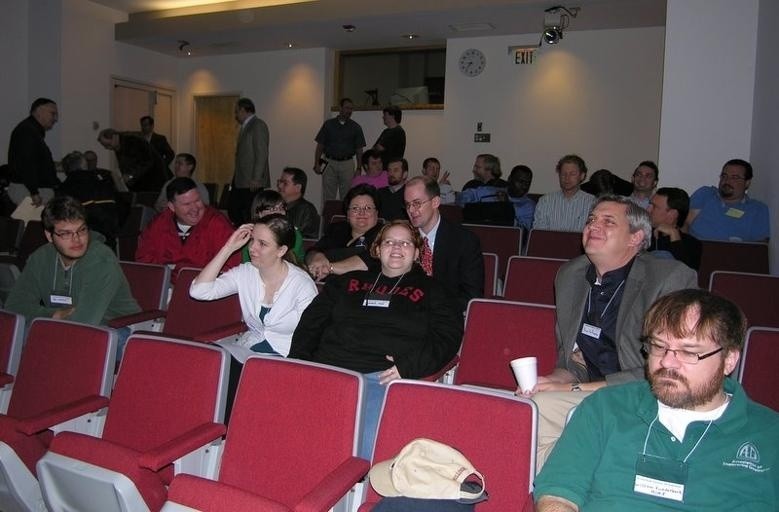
[1,180,779,512]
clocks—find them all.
[456,48,487,78]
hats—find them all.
[369,438,485,500]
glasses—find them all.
[642,335,723,364]
[406,198,432,210]
[380,239,413,247]
[719,174,746,182]
[50,226,87,240]
[347,206,377,212]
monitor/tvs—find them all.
[390,86,428,105]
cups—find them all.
[511,355,540,392]
